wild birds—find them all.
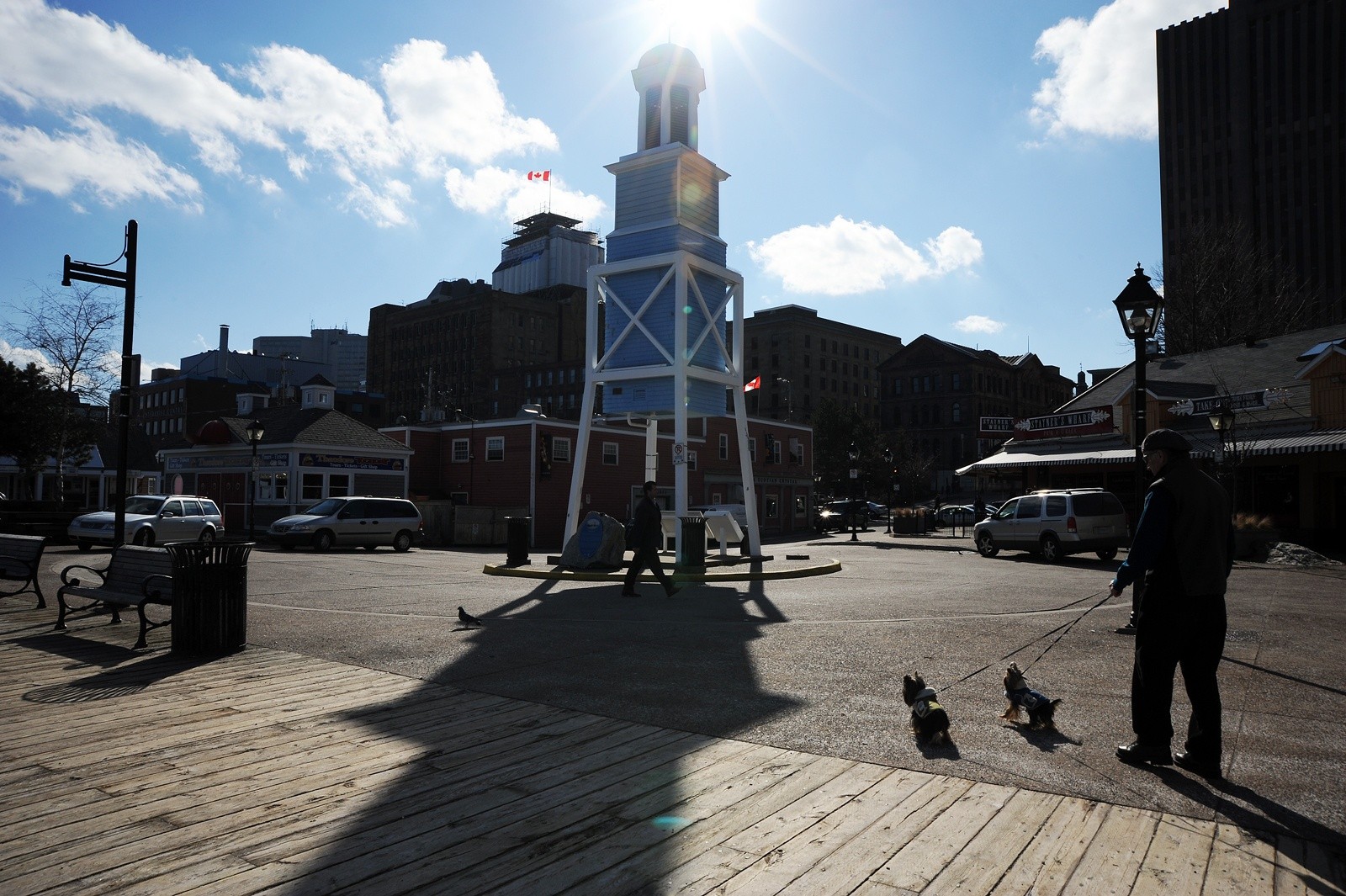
[457,606,485,628]
[959,551,963,555]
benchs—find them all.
[54,542,215,650]
[0,533,47,610]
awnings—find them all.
[955,425,1346,475]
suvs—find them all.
[270,495,427,554]
[972,487,1133,562]
[69,493,225,552]
[812,496,887,533]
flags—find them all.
[528,170,549,181]
[744,376,761,392]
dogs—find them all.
[902,670,950,737]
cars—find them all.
[933,503,999,524]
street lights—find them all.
[813,472,822,514]
[844,440,860,540]
[246,418,264,547]
[1112,261,1166,527]
[1207,395,1235,568]
[881,448,893,534]
[455,410,478,505]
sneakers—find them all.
[1115,740,1173,765]
[1173,752,1221,779]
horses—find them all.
[1002,661,1063,730]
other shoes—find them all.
[621,589,641,597]
[665,585,684,597]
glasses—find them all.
[1143,450,1165,464]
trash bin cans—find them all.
[740,526,763,554]
[506,517,532,567]
[677,517,709,566]
[165,541,253,655]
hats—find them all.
[1141,429,1195,454]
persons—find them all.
[1026,488,1033,495]
[1109,428,1233,772]
[974,498,985,511]
[934,494,941,512]
[622,481,683,599]
